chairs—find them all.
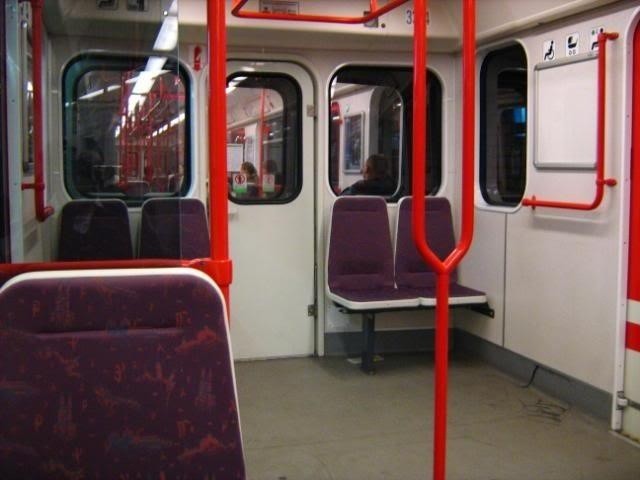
[53,198,134,261]
[136,197,210,262]
[328,193,491,378]
[1,267,245,479]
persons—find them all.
[76,136,121,188]
[234,161,259,201]
[263,158,283,200]
[143,165,179,194]
[337,154,408,203]
